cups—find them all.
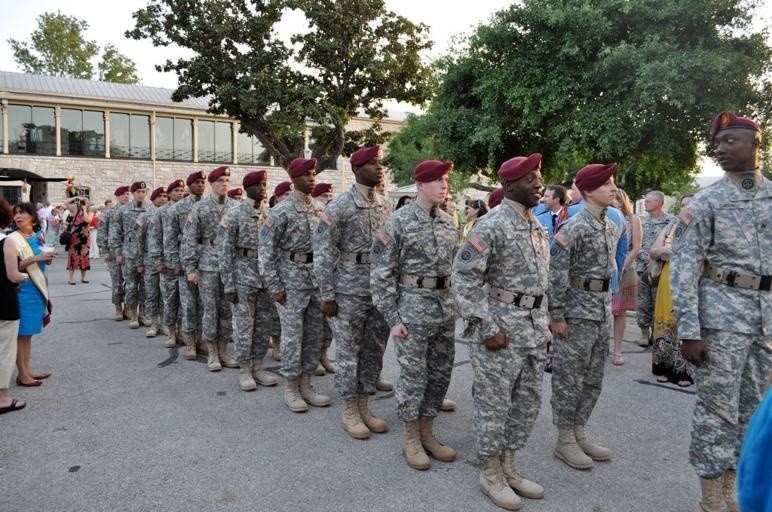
[40,245,54,253]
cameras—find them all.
[80,200,86,205]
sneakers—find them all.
[67,280,77,286]
[676,377,693,387]
[81,279,90,284]
[612,350,626,366]
[656,375,670,383]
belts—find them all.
[234,247,258,259]
[200,237,218,246]
[122,237,131,243]
[569,275,612,292]
[397,272,454,289]
[487,284,549,309]
[701,266,772,292]
[279,247,314,264]
[339,251,371,265]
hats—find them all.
[574,161,618,194]
[496,153,544,182]
[149,185,165,202]
[130,180,147,194]
[186,170,207,186]
[412,158,454,183]
[242,169,268,187]
[227,187,242,199]
[207,165,231,184]
[349,144,382,168]
[114,185,130,198]
[274,181,295,197]
[487,187,505,210]
[166,179,186,194]
[310,182,335,199]
[710,111,759,145]
[287,156,317,178]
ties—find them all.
[551,213,558,226]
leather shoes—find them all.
[15,377,44,387]
[28,369,54,380]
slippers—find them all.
[1,395,25,416]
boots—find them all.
[552,424,593,471]
[574,423,615,461]
[638,325,655,348]
[357,393,389,432]
[499,447,545,500]
[477,451,523,510]
[368,375,394,395]
[439,396,458,412]
[112,294,338,413]
[399,415,432,471]
[419,412,457,463]
[339,398,372,440]
[721,466,742,512]
[694,472,730,511]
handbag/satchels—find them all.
[59,227,72,246]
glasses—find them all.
[465,206,470,210]
